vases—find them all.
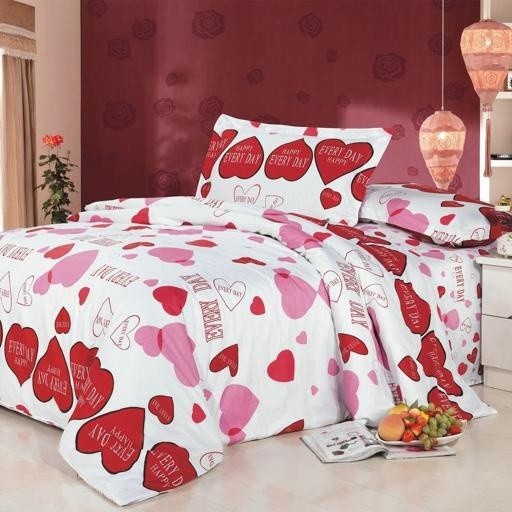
[51,210,65,223]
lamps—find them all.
[419,1,512,191]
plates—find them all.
[374,418,468,445]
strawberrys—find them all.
[402,408,425,442]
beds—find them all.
[1,193,498,501]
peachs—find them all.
[378,414,405,441]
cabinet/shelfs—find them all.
[476,1,512,393]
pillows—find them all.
[359,182,511,249]
[194,113,393,229]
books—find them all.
[298,418,457,464]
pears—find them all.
[387,403,409,415]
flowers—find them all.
[35,134,79,217]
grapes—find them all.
[418,403,462,451]
